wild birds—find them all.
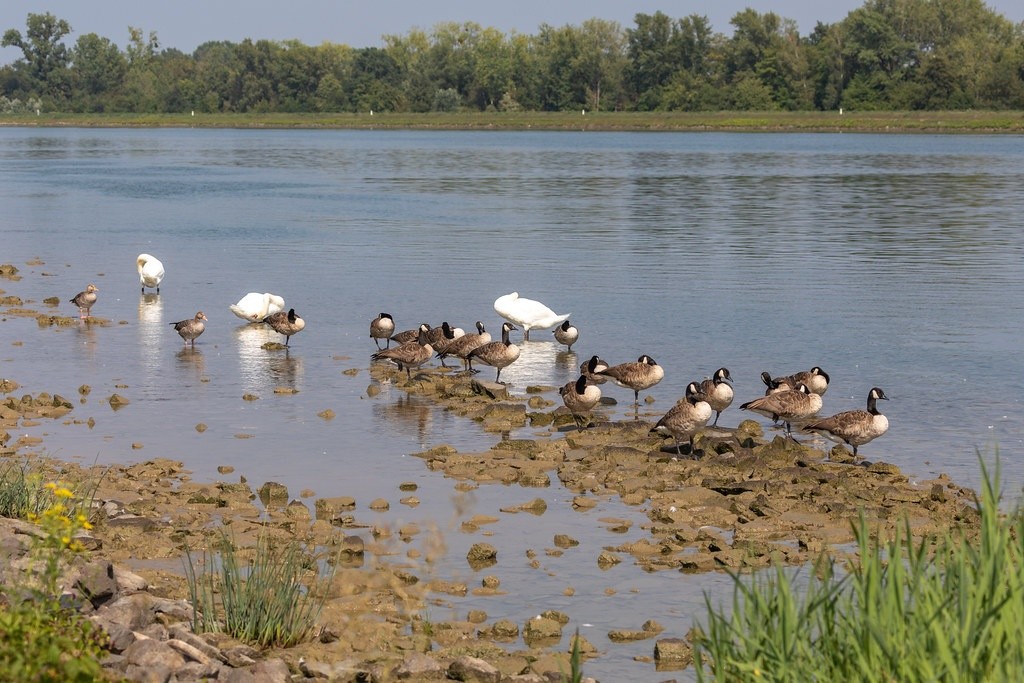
[650,368,734,460]
[739,367,889,457]
[137,254,166,292]
[370,313,520,385]
[554,320,664,427]
[494,291,572,340]
[169,311,208,346]
[230,292,285,323]
[69,285,99,319]
[263,309,304,347]
